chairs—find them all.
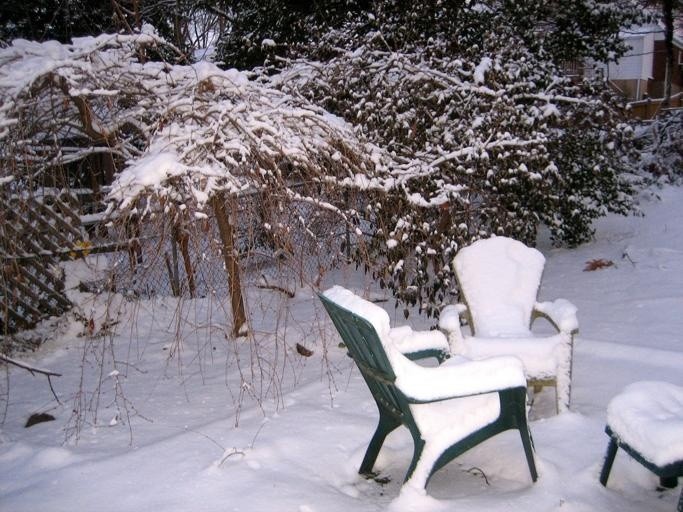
[599,426,683,512]
[438,236,579,415]
[316,292,538,492]
